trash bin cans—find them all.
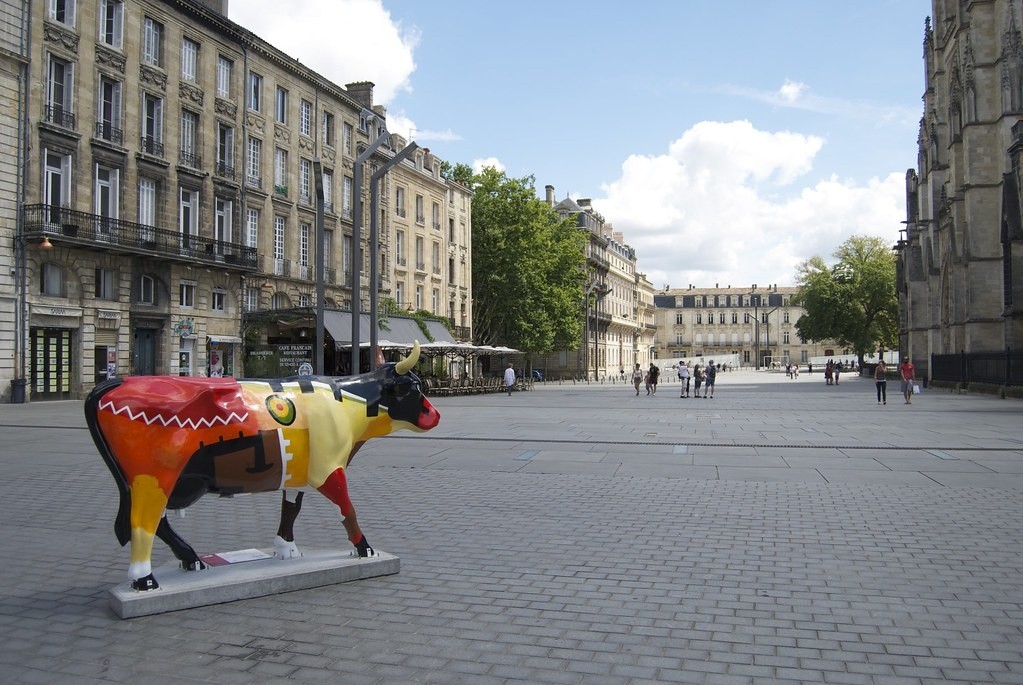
[863,366,869,376]
[10,378,27,404]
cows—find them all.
[84,339,442,593]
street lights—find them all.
[747,313,760,372]
[595,288,614,381]
[369,140,420,372]
[765,304,782,370]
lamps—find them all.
[447,310,467,317]
[300,327,308,337]
[13,234,52,252]
[248,277,272,288]
[396,302,414,311]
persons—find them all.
[644,363,660,396]
[631,363,643,396]
[786,363,798,379]
[809,363,812,375]
[693,360,715,398]
[679,360,692,398]
[900,356,915,404]
[828,359,858,368]
[875,362,887,405]
[717,362,732,372]
[825,362,839,385]
[504,364,515,396]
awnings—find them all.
[206,336,242,350]
[313,309,458,351]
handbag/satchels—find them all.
[912,382,920,394]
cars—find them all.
[516,369,543,382]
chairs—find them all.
[423,376,534,397]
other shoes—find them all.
[680,393,714,399]
[905,399,912,404]
[878,401,882,404]
[883,401,886,405]
[636,391,656,396]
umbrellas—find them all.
[341,340,527,378]
